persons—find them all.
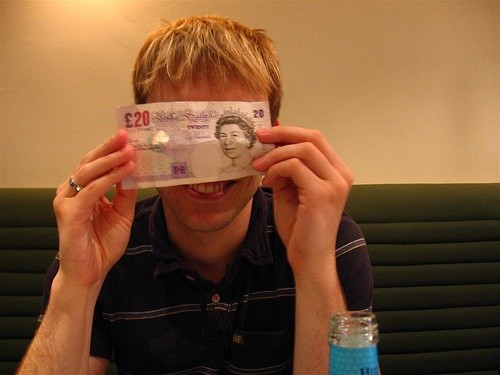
[16,15,375,373]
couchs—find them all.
[2,179,499,374]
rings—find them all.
[68,175,83,191]
